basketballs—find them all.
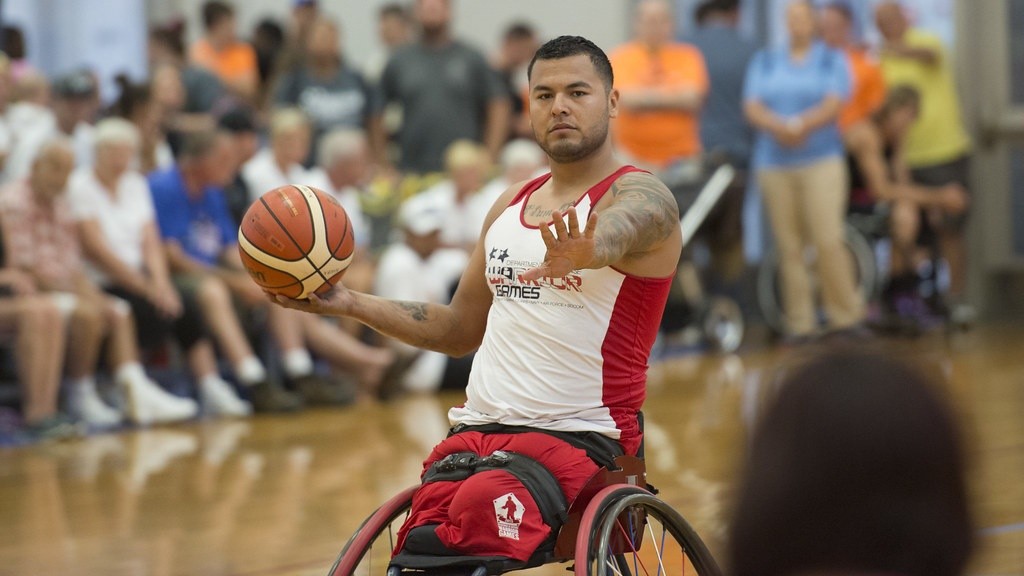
[236,182,357,300]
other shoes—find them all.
[369,346,423,400]
[204,385,248,415]
[775,330,853,348]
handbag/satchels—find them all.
[868,269,931,339]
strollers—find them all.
[661,162,748,354]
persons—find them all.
[726,354,975,576]
[266,38,683,558]
[0,0,985,444]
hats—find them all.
[50,73,89,97]
[155,14,185,24]
[404,193,440,237]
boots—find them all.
[114,362,195,423]
[70,378,121,426]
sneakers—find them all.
[239,363,350,410]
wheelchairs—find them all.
[758,222,982,340]
[328,411,723,576]
